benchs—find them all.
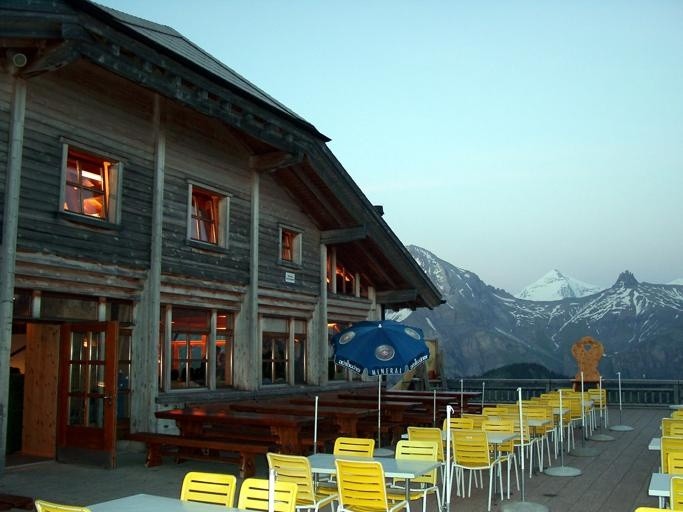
[124,431,268,479]
[0,493,34,512]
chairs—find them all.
[155,381,608,512]
[633,409,682,512]
[34,499,91,512]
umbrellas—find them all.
[330,318,432,448]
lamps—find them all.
[31,290,41,319]
[96,297,106,322]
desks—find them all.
[83,494,265,512]
